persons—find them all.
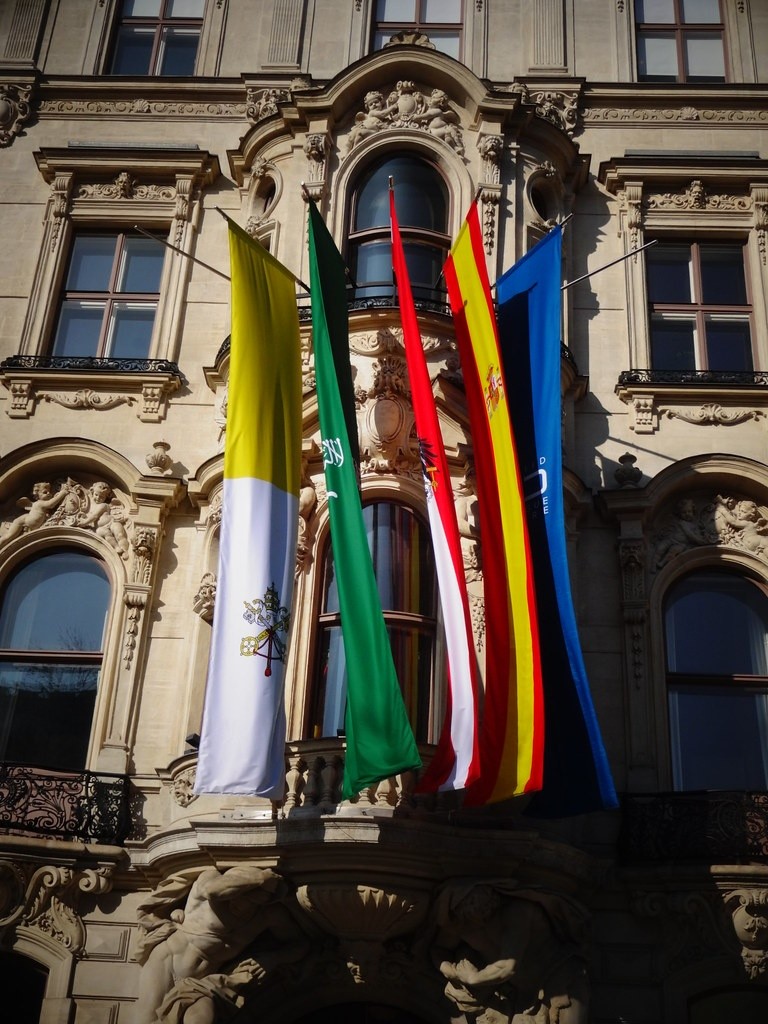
[409,89,465,160]
[434,882,594,1024]
[649,496,708,573]
[716,499,768,562]
[77,480,129,561]
[0,482,69,549]
[124,865,285,1024]
[339,91,398,160]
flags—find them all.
[305,205,424,803]
[390,192,620,821]
[191,223,301,799]
